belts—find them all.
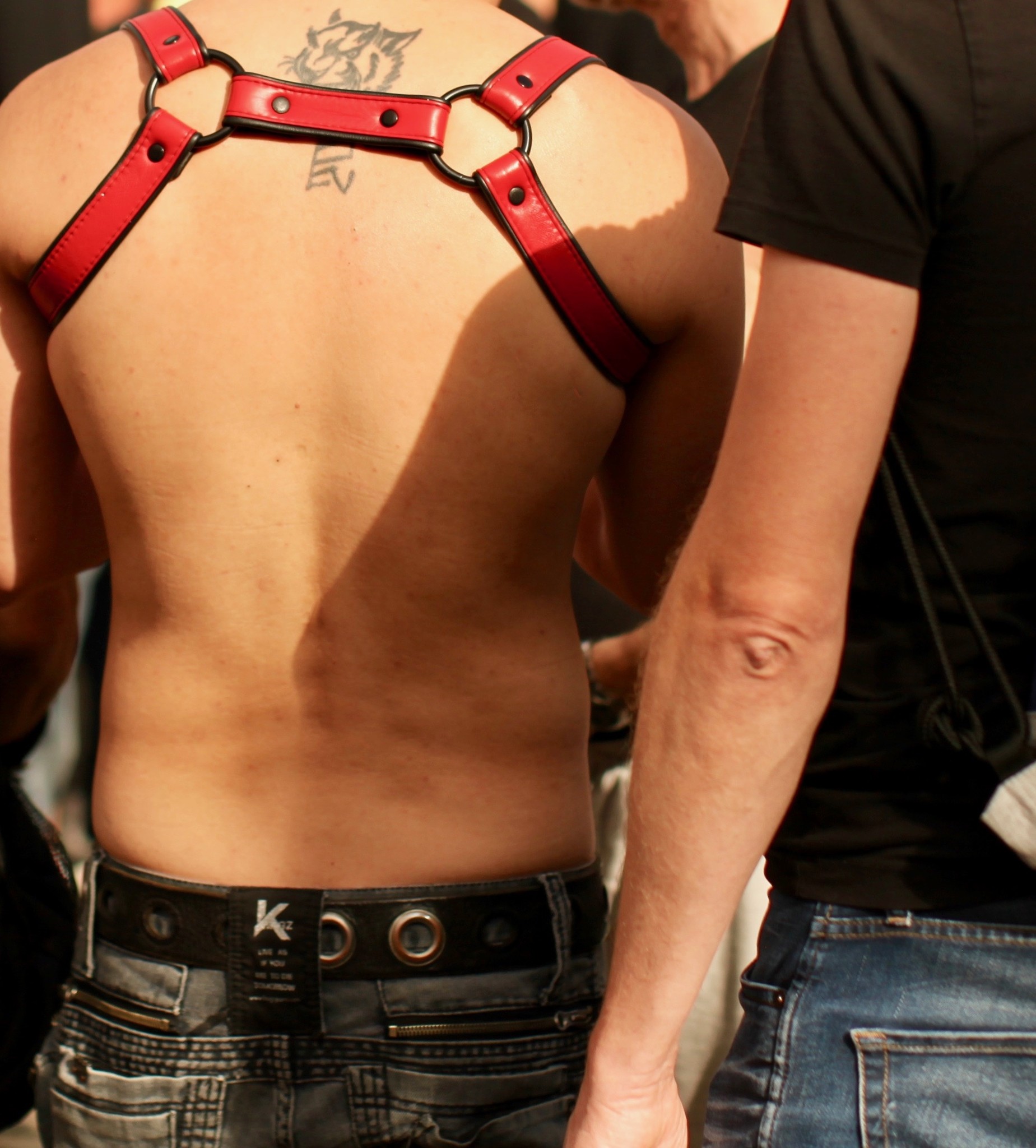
[92,862,609,982]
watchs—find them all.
[578,639,629,732]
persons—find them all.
[0,3,1036,1146]
